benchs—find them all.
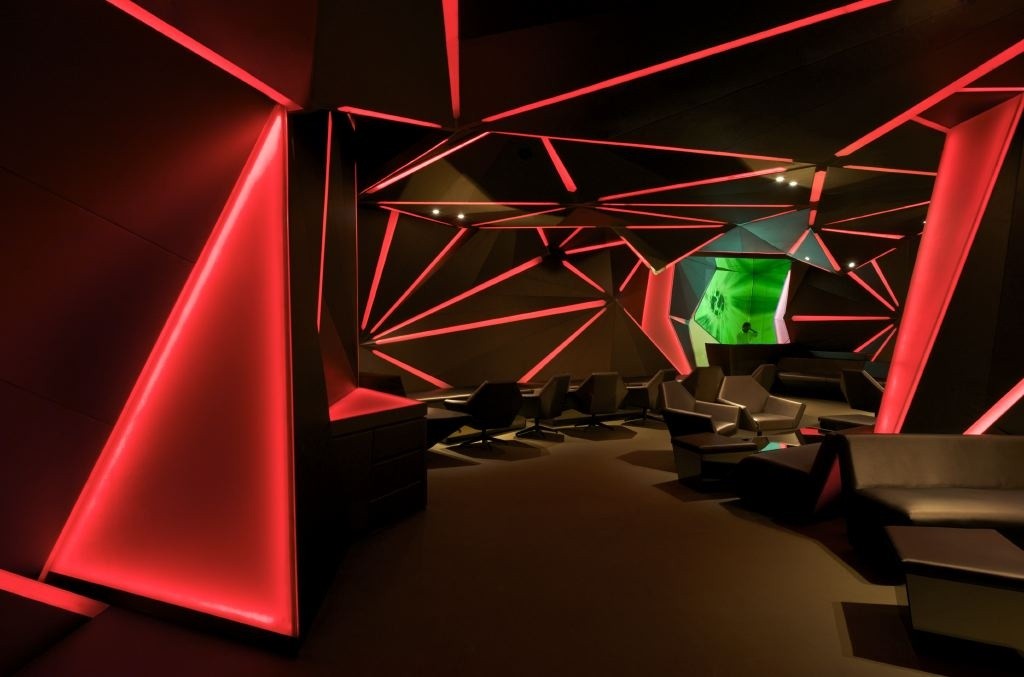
[776,357,889,400]
[817,414,876,434]
[741,427,1024,557]
[671,431,758,492]
[883,524,1024,653]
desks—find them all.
[405,375,651,443]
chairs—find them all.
[359,373,405,397]
[444,363,808,451]
